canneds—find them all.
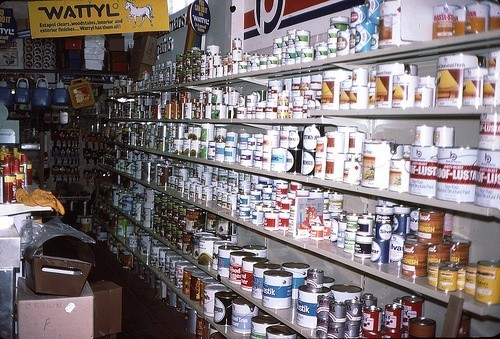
[98,0,500,339]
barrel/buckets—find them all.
[68,77,95,109]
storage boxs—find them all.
[65,32,158,80]
[12,253,124,339]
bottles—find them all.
[0,146,32,204]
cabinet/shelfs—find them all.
[0,31,500,339]
